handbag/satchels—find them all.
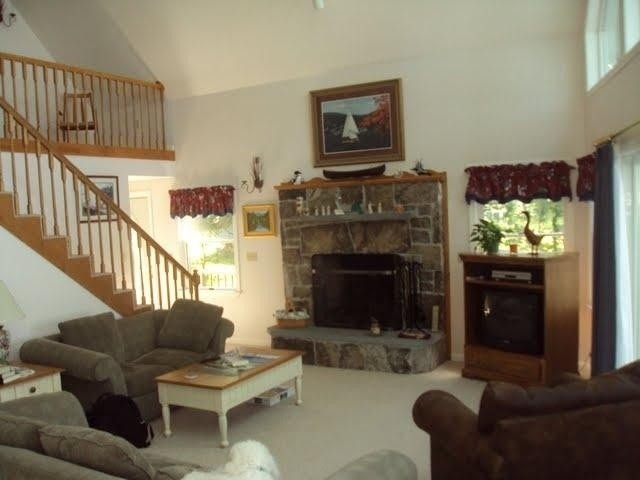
[87,391,156,452]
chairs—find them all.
[61,93,98,144]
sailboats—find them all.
[340,111,360,142]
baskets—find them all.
[274,300,308,329]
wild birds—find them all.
[520,210,545,256]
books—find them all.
[0,363,21,384]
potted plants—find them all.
[470,220,505,254]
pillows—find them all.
[157,297,224,352]
[58,313,125,364]
[0,409,43,456]
[39,425,156,480]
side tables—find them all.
[0,362,66,402]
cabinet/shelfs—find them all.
[461,252,579,385]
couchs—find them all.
[413,360,640,480]
[20,309,234,422]
[0,392,418,480]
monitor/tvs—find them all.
[480,288,543,354]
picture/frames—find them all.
[77,175,119,223]
[243,204,277,237]
[310,77,405,167]
